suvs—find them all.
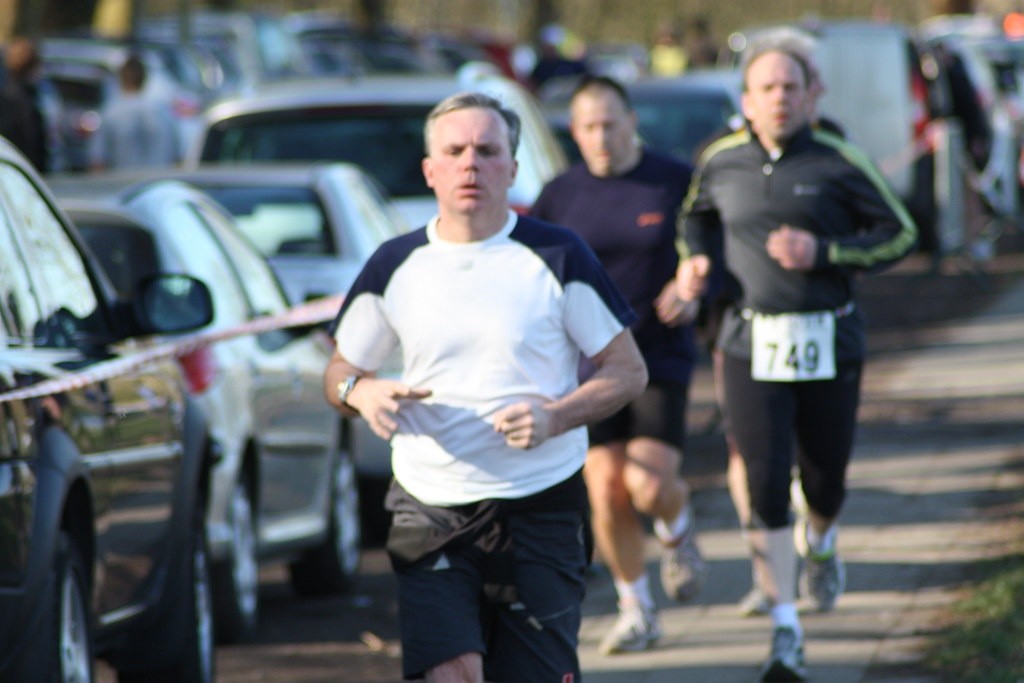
[191,59,558,282]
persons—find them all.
[0,39,51,172]
[87,55,184,176]
[322,93,649,683]
[648,12,718,75]
[513,24,594,98]
[524,74,710,653]
[674,46,918,683]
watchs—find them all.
[336,374,363,414]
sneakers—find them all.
[651,503,706,603]
[792,508,846,614]
[757,624,809,683]
[599,593,662,655]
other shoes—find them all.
[736,588,775,617]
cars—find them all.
[1,10,1024,326]
[114,158,406,549]
[0,140,229,683]
[45,172,371,638]
